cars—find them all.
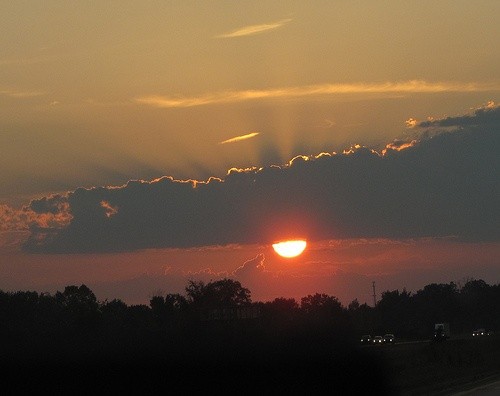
[373,336,384,345]
[472,328,495,338]
[384,334,395,344]
[359,335,372,345]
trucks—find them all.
[434,323,450,340]
